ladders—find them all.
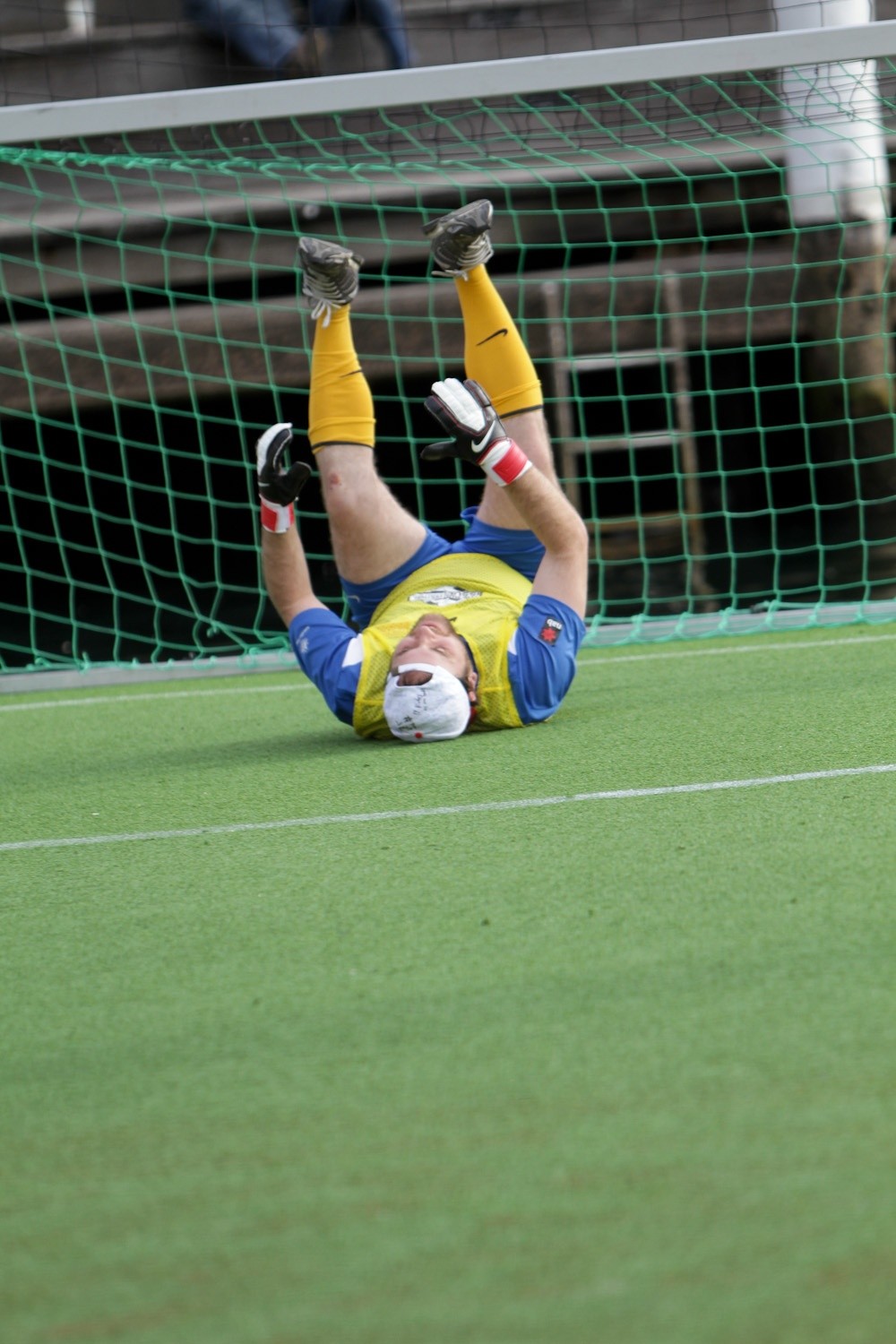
[541,270,721,613]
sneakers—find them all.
[296,237,363,328]
[421,200,496,282]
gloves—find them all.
[419,377,533,487]
[255,422,312,535]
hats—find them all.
[383,662,471,744]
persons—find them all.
[188,0,416,81]
[255,198,590,743]
